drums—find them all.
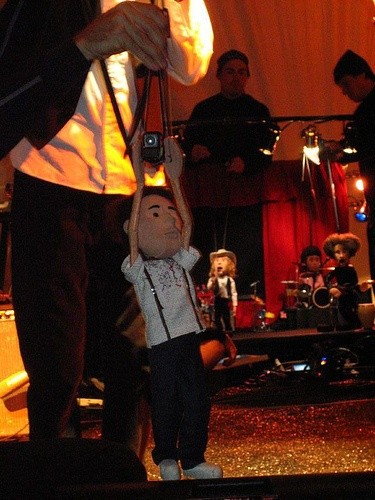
[297,283,311,296]
[312,286,334,309]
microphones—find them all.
[250,281,260,288]
[319,257,330,267]
[293,261,306,267]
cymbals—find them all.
[320,266,336,271]
[281,280,298,284]
[292,261,306,267]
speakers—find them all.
[317,320,334,332]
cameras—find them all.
[142,131,166,163]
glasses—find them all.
[340,76,355,89]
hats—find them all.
[332,48,370,75]
[217,49,248,66]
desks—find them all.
[232,327,371,391]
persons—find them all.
[182,47,277,302]
[0,0,168,453]
[121,144,223,481]
[314,46,375,296]
[322,232,366,331]
[206,250,237,333]
[297,245,323,290]
